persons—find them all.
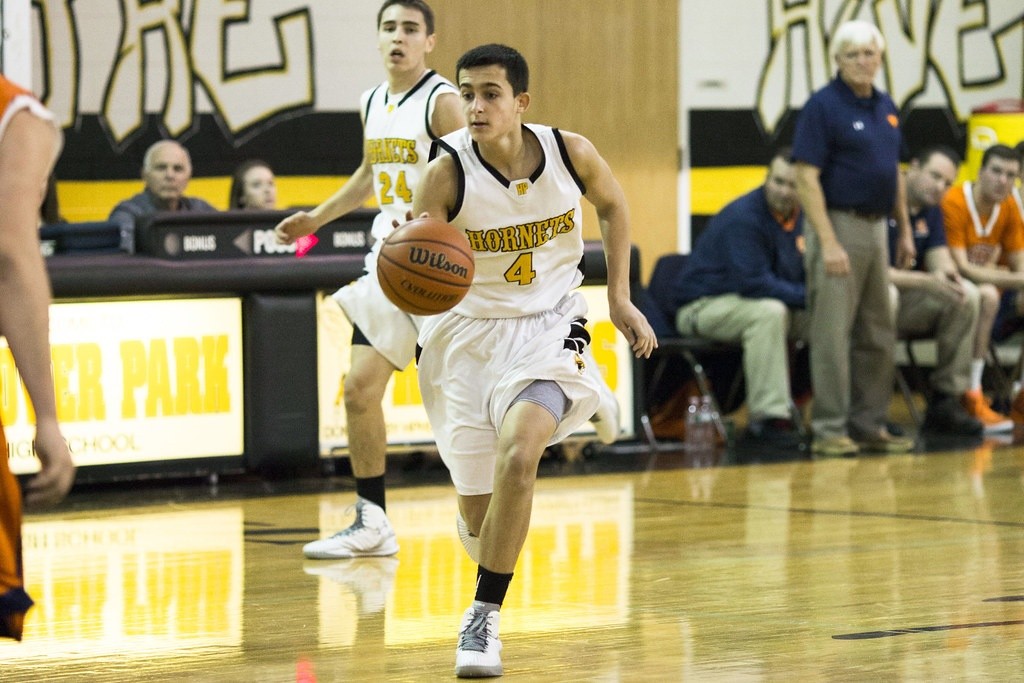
[108,139,218,256]
[787,20,917,458]
[276,0,472,560]
[669,146,812,449]
[381,44,658,676]
[0,75,76,644]
[886,140,1024,435]
[229,161,277,214]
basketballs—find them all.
[376,217,475,315]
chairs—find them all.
[894,327,938,430]
[649,254,737,445]
[629,247,728,453]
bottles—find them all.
[684,395,716,455]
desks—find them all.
[0,239,635,496]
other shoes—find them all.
[731,418,813,462]
[922,390,985,451]
[811,431,858,455]
[966,389,1017,434]
[850,422,914,451]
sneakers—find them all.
[303,495,399,559]
[454,600,502,676]
[456,507,480,563]
